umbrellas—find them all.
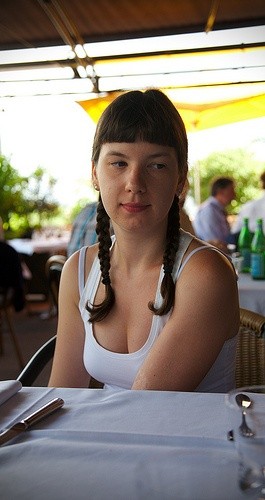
[76,80,265,202]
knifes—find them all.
[0,398,64,448]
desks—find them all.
[0,386,265,500]
[228,269,265,314]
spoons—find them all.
[235,394,254,438]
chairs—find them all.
[17,305,265,398]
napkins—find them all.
[0,376,22,409]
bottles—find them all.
[250,219,265,279]
[238,219,254,273]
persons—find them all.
[232,172,265,234]
[196,176,238,253]
[48,89,240,392]
[67,202,115,261]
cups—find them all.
[225,382,265,500]
